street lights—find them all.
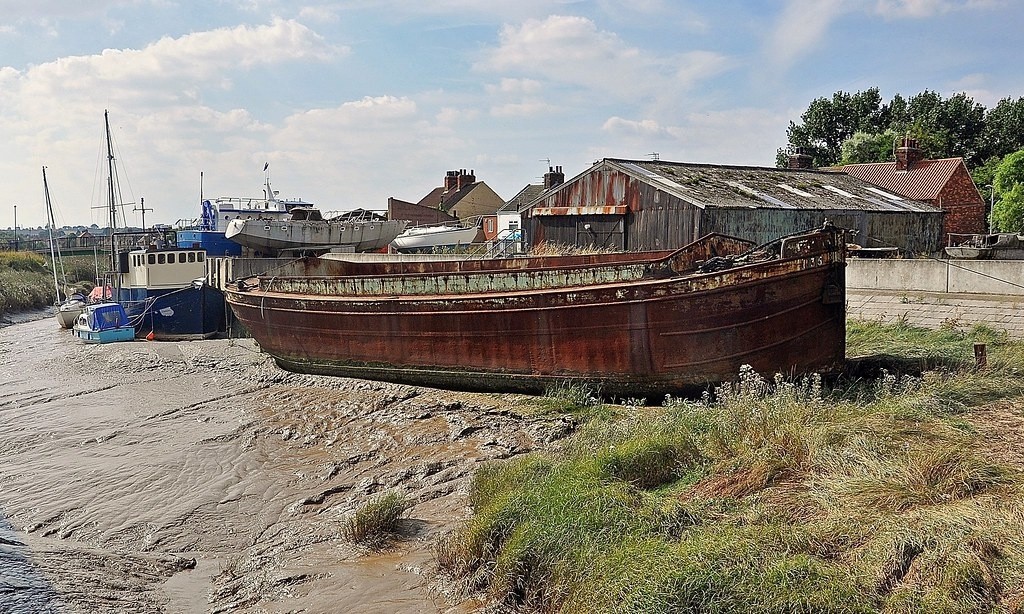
[985,185,994,234]
[14,206,16,240]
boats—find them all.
[41,110,478,345]
[225,221,845,396]
